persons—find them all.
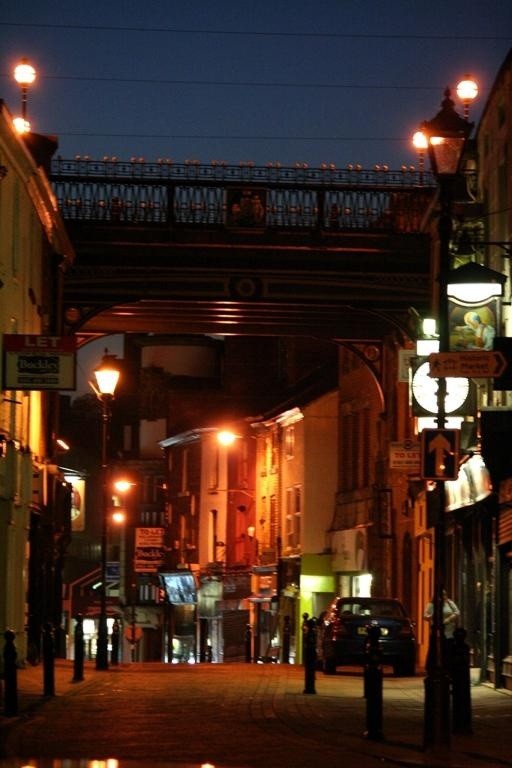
[452,310,495,352]
[424,588,461,677]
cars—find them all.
[316,596,416,677]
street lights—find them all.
[418,84,476,743]
[94,346,124,672]
[218,420,288,666]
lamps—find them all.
[435,240,512,308]
[413,121,443,186]
[14,55,36,120]
[455,73,479,119]
[417,314,440,340]
[12,117,31,135]
